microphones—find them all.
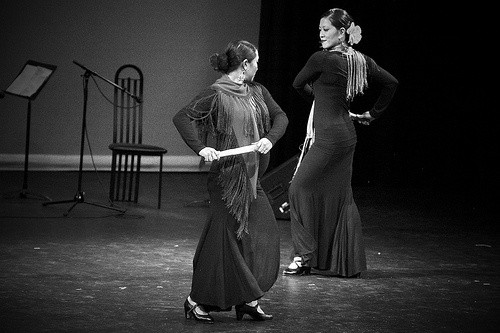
[134,96,143,103]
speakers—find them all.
[259,154,300,219]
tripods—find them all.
[0,99,53,202]
[42,60,137,216]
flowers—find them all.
[347,22,363,46]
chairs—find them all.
[108,64,169,210]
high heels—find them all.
[235,301,273,322]
[284,258,312,275]
[183,298,214,324]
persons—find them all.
[282,7,398,277]
[173,38,289,323]
